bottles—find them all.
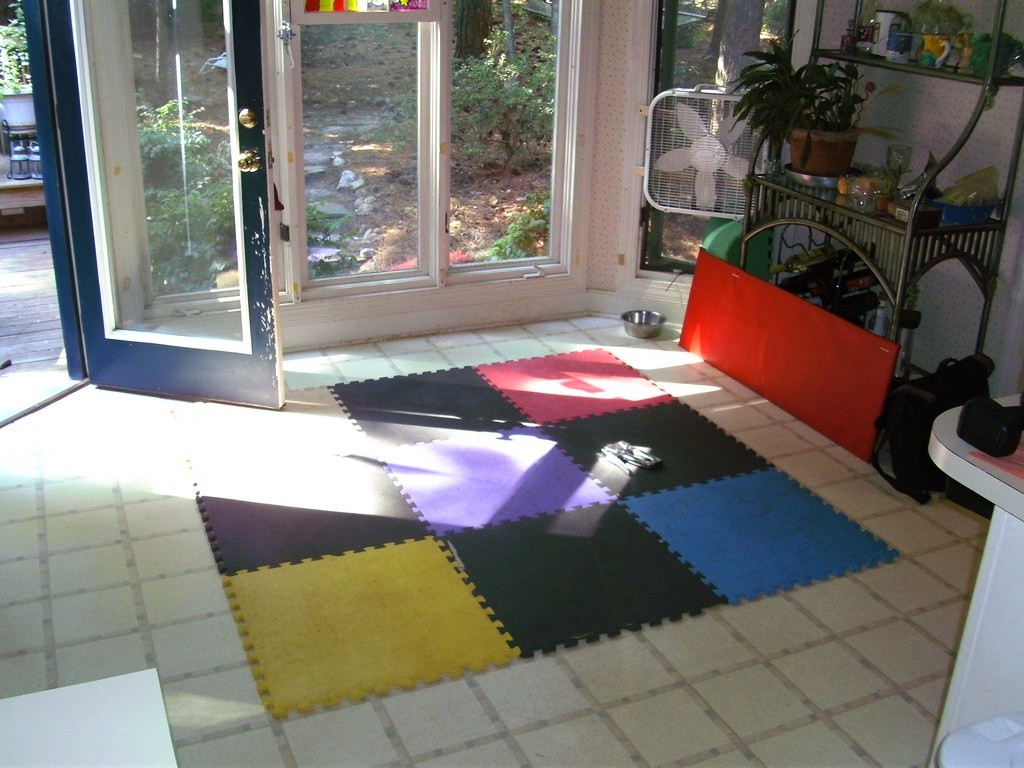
[768,244,833,275]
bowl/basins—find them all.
[619,309,667,339]
[931,197,999,226]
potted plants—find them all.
[857,0,883,58]
[0,0,37,130]
[966,31,1023,78]
[886,15,912,64]
[911,0,969,69]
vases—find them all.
[784,121,860,177]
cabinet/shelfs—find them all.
[928,392,1024,768]
[741,0,1024,390]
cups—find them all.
[885,33,952,69]
[844,162,889,214]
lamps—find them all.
[27,138,43,180]
[2,119,30,180]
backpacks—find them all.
[874,353,995,506]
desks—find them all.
[0,153,47,215]
[0,667,180,768]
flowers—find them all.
[727,30,877,144]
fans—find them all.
[642,84,771,221]
